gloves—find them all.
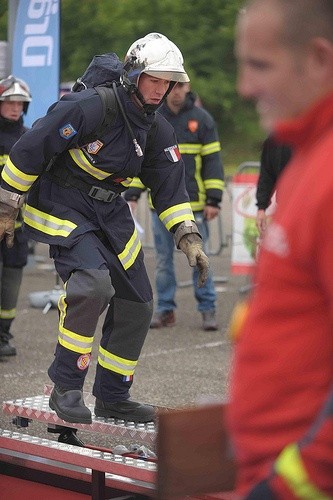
[0,201,19,250]
[179,232,209,288]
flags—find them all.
[12,0,60,131]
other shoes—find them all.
[150,310,176,328]
[0,340,15,354]
[202,310,218,330]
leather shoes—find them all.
[49,384,92,425]
[94,396,154,422]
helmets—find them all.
[0,74,33,114]
[124,32,189,89]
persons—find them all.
[124,74,224,330]
[234,0,333,500]
[0,74,32,355]
[0,32,209,424]
[254,130,294,231]
